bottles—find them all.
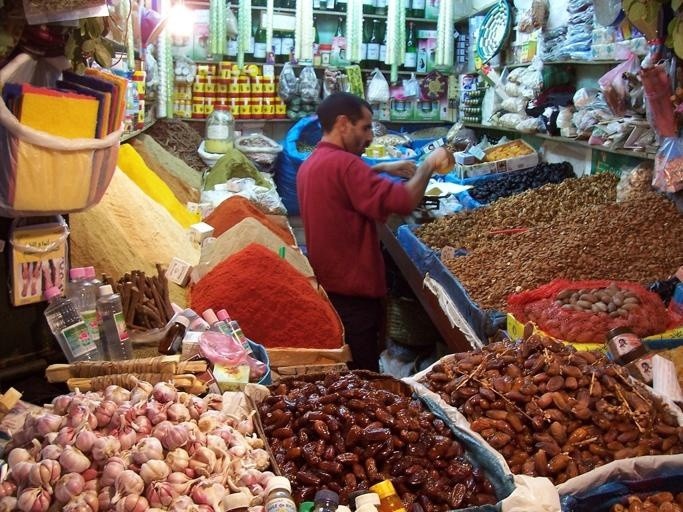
[224,0,424,70]
[157,305,255,369]
[224,478,404,511]
[44,266,134,365]
[462,91,484,125]
[205,104,235,153]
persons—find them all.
[293,90,456,374]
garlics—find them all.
[0,376,275,512]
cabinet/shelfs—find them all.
[167,0,448,124]
[460,56,653,159]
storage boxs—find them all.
[453,137,538,181]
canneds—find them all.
[319,44,331,67]
[192,60,287,120]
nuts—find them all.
[132,70,146,129]
